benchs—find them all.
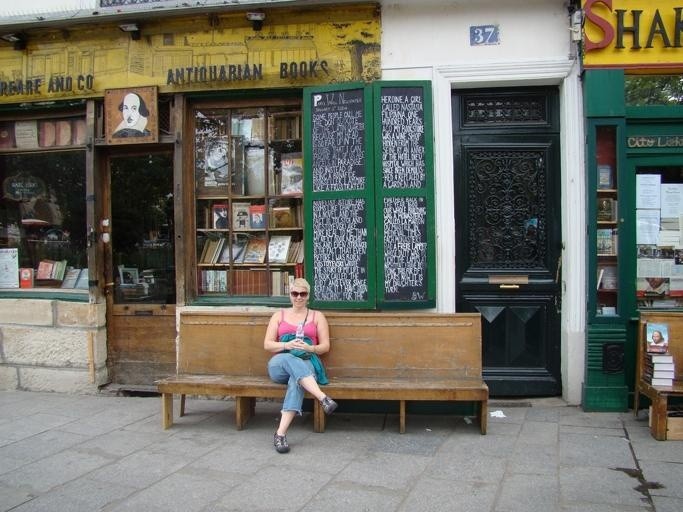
[153,311,489,436]
[633,311,683,442]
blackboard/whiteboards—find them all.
[303,81,434,309]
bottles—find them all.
[295,321,304,343]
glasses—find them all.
[290,291,308,298]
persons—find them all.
[111,92,151,136]
[264,278,339,454]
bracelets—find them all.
[284,341,287,352]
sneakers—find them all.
[320,395,338,415]
[274,432,290,453]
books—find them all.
[19,257,89,290]
[195,111,306,297]
[594,162,618,316]
[0,117,86,148]
[642,321,675,387]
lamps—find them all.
[118,22,142,39]
[1,33,28,50]
[246,11,266,31]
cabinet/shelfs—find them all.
[195,107,304,297]
[596,187,617,294]
